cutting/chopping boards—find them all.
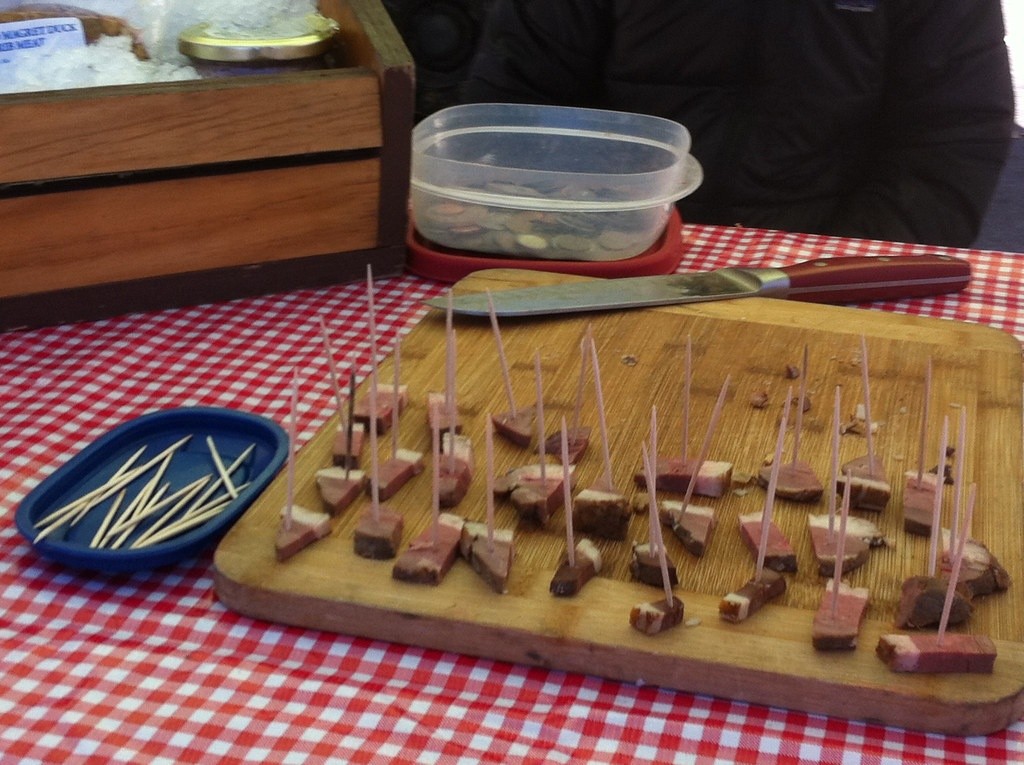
[214,264,1023,738]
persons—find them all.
[384,0,1015,248]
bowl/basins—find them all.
[407,98,703,260]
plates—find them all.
[15,407,289,571]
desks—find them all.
[0,222,1024,765]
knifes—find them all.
[421,252,974,321]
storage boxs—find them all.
[0,0,415,333]
